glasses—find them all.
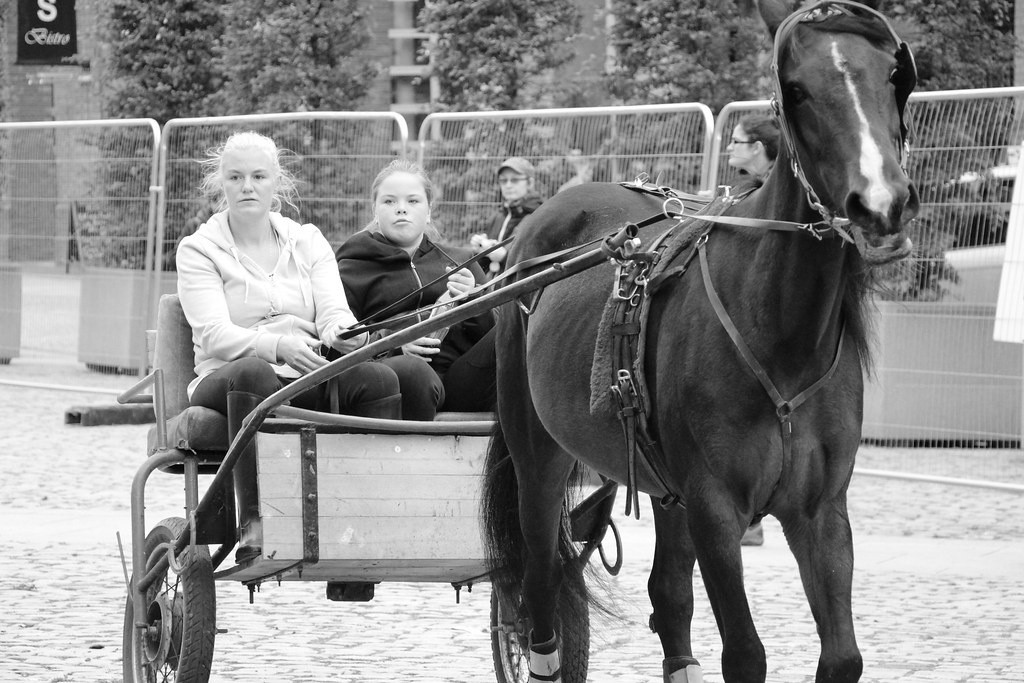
[498,177,528,184]
[730,137,753,145]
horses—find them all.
[483,0,918,683]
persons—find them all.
[339,157,503,421]
[728,113,782,181]
[473,156,544,275]
[176,127,403,569]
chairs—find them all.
[146,291,229,472]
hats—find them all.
[498,157,535,175]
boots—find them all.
[222,389,270,563]
[349,393,401,419]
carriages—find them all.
[65,0,943,683]
